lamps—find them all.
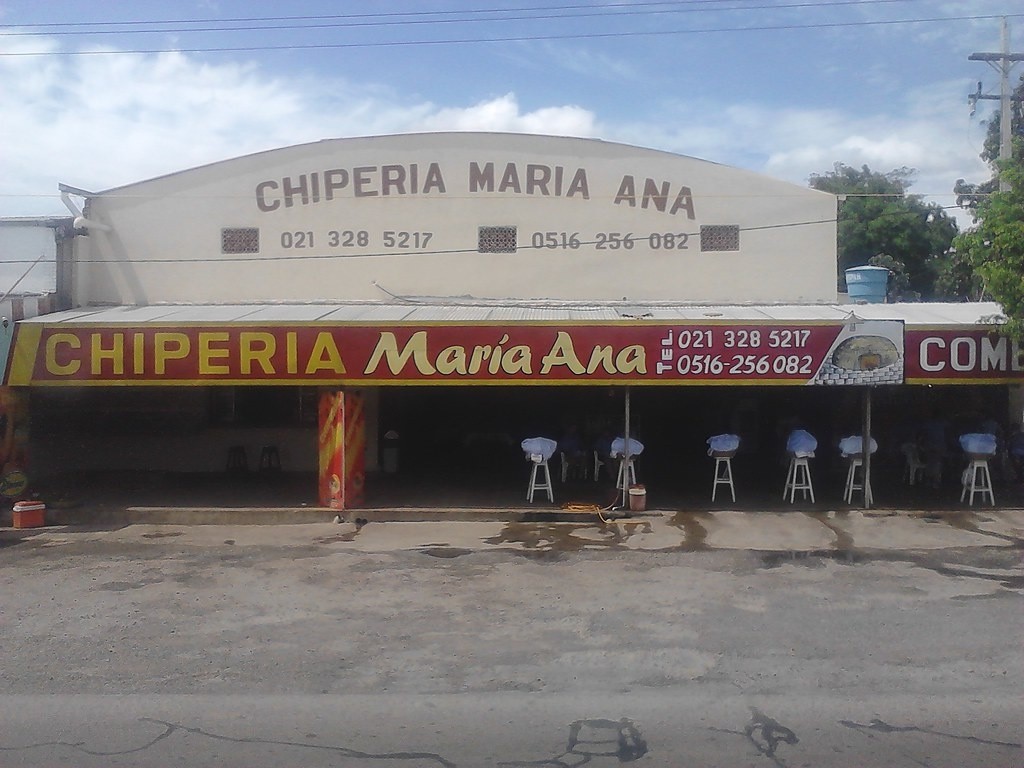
[843,310,864,332]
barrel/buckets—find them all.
[629,487,646,511]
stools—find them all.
[526,461,554,504]
[616,458,637,489]
[843,460,872,505]
[712,456,736,502]
[260,446,281,482]
[224,447,250,480]
[783,459,816,503]
[959,460,995,507]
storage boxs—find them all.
[12,501,45,528]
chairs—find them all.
[900,443,927,485]
